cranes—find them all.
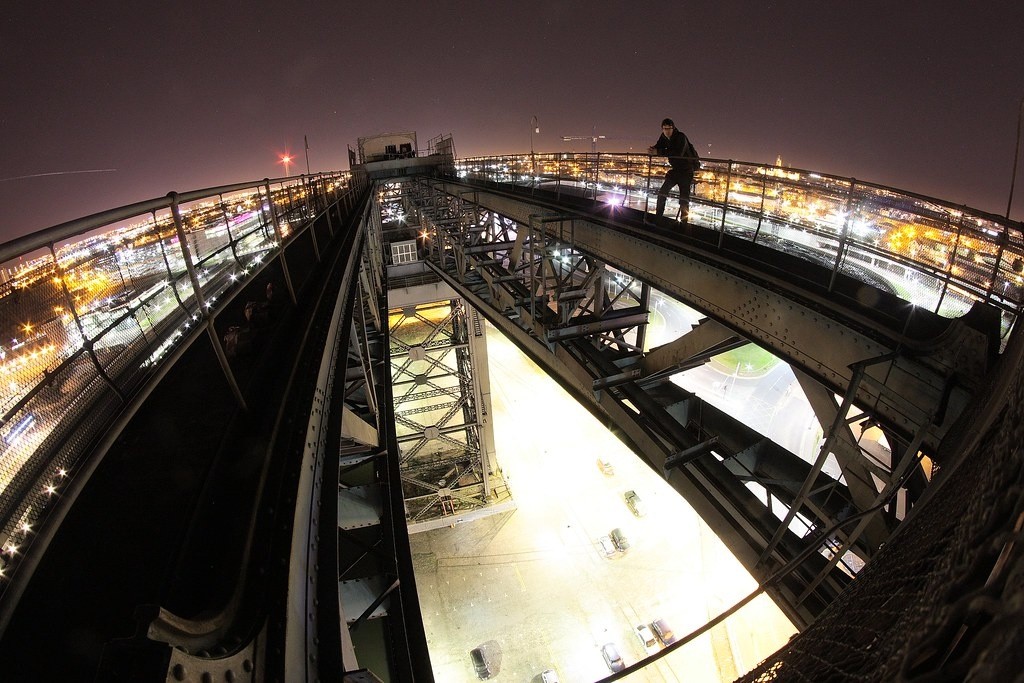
[558,121,605,178]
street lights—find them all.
[530,115,539,181]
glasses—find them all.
[663,128,674,130]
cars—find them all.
[470,452,677,683]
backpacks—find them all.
[680,132,700,171]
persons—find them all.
[648,118,694,230]
